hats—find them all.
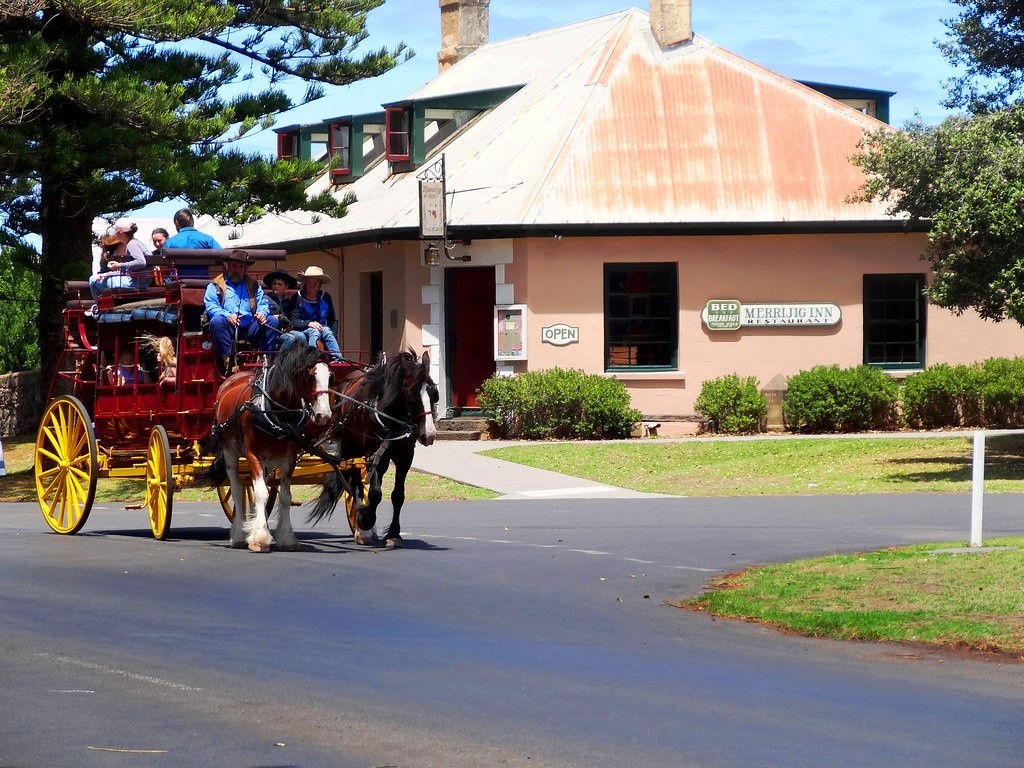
[114,218,137,234]
[221,249,256,265]
[297,264,333,284]
[263,269,297,287]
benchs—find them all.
[63,281,96,309]
[98,256,172,310]
[162,249,286,306]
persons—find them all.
[84,209,223,319]
[204,249,346,378]
[105,335,178,386]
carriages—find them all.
[35,250,439,552]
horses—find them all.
[215,338,334,552]
[303,342,439,548]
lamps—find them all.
[422,237,443,267]
[374,238,382,248]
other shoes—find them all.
[221,355,239,378]
